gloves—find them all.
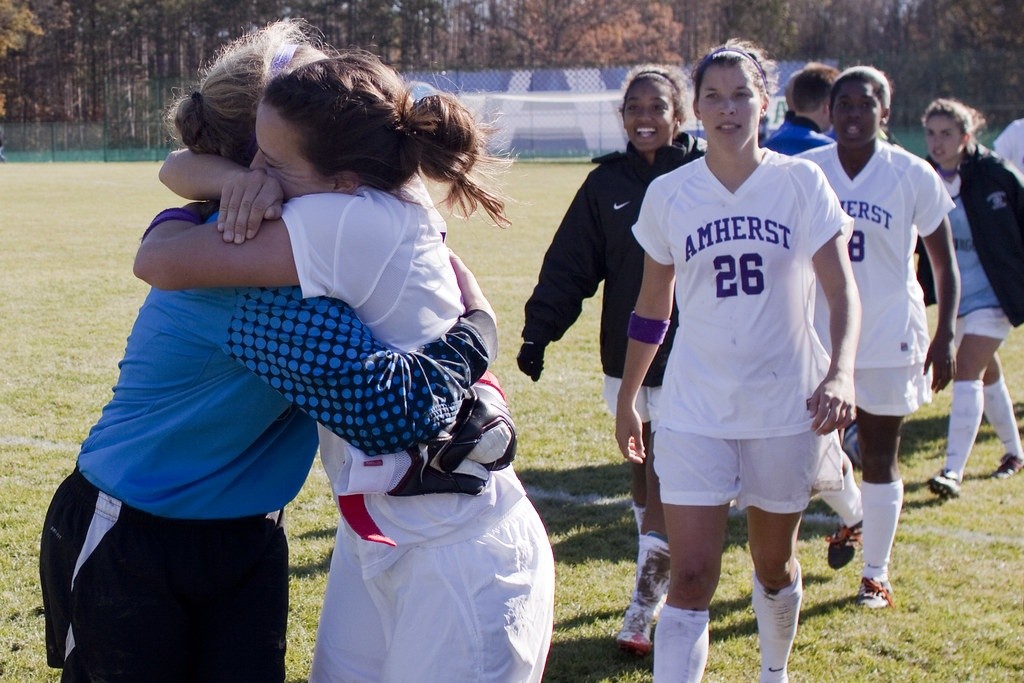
[332,370,517,548]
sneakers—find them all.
[996,453,1024,479]
[826,521,863,571]
[856,575,895,609]
[928,468,961,499]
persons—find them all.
[915,98,1024,497]
[614,47,862,683]
[992,116,1024,177]
[517,63,708,656]
[133,51,553,683]
[792,65,961,609]
[41,19,499,683]
[755,62,906,470]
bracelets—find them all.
[141,208,198,242]
[626,311,670,344]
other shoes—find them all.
[617,630,652,653]
